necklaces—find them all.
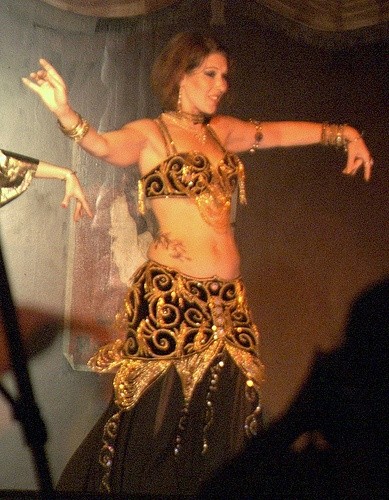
[161,109,212,143]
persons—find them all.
[0,149,95,224]
[20,24,373,495]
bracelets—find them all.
[314,121,363,153]
[58,109,88,144]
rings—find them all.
[364,159,373,165]
